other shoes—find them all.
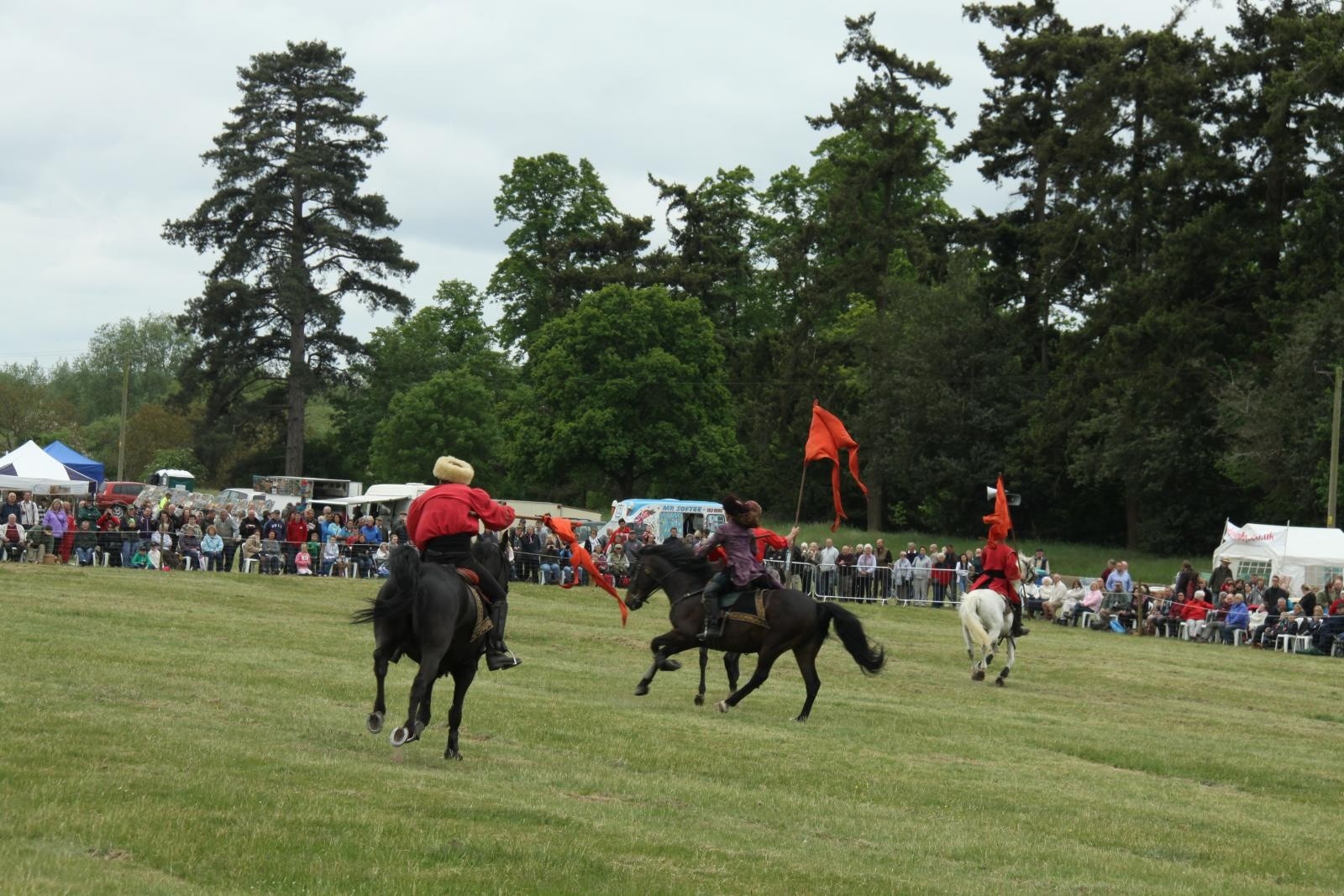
[1250,644,1262,649]
[274,570,280,574]
[79,561,88,566]
[1064,614,1072,619]
[1196,636,1207,642]
[1092,622,1101,627]
[1057,620,1066,625]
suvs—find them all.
[96,481,149,519]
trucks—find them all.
[215,473,609,546]
[150,469,195,492]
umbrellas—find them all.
[0,439,105,506]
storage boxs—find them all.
[45,557,57,564]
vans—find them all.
[576,498,727,551]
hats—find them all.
[140,546,148,551]
[1221,558,1231,564]
[127,504,134,510]
[83,496,95,502]
[375,517,383,521]
[629,530,635,535]
[1036,548,1043,552]
[520,518,526,523]
[748,500,762,516]
[433,455,474,486]
[399,512,407,516]
[724,495,747,515]
[261,511,272,516]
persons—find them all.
[970,524,1031,637]
[406,454,523,671]
[763,538,983,608]
[471,512,725,586]
[0,490,406,577]
[1019,548,1344,655]
[693,495,800,645]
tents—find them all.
[1212,516,1344,601]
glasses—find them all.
[616,548,622,550]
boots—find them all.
[697,595,724,641]
[485,601,520,670]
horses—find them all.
[958,551,1037,685]
[348,526,509,761]
[620,537,889,723]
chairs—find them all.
[101,552,123,568]
[349,546,371,578]
[537,555,562,585]
[242,542,260,573]
[1019,584,1328,654]
[328,554,351,576]
[561,568,583,586]
[181,554,209,571]
[75,551,96,567]
